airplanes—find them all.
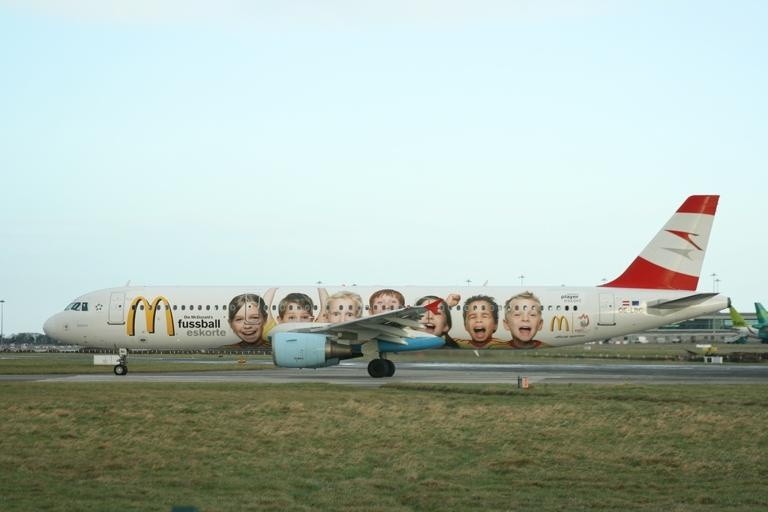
[724,302,768,344]
[42,194,732,379]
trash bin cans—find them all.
[690,336,697,343]
[519,377,529,388]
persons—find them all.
[413,296,461,349]
[457,295,506,349]
[705,351,718,362]
[261,288,329,343]
[367,289,405,315]
[214,294,272,355]
[325,291,363,322]
[488,292,548,349]
